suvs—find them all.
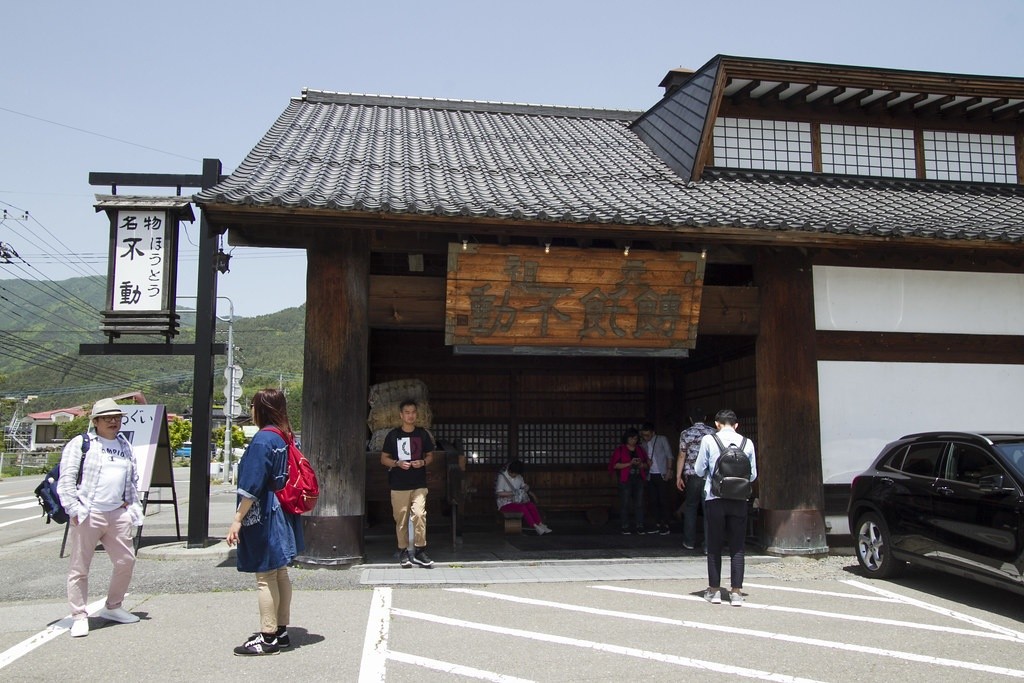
[847,432,1024,599]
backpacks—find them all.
[34,433,90,524]
[707,433,753,501]
[612,446,623,486]
[260,428,319,514]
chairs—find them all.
[904,458,934,477]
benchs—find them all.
[503,512,523,533]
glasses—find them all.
[98,415,122,422]
[250,401,255,409]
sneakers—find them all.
[399,550,413,566]
[99,607,141,623]
[248,629,291,648]
[71,617,89,636]
[413,549,434,566]
[233,634,281,655]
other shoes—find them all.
[534,526,545,535]
[542,525,552,533]
[647,525,660,534]
[682,541,694,550]
[704,587,721,604]
[728,592,742,606]
[620,527,631,534]
[701,542,709,555]
[659,525,670,535]
[637,526,646,535]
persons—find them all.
[495,462,552,536]
[56,398,140,638]
[610,429,649,535]
[641,426,673,533]
[226,389,305,656]
[380,401,434,568]
[695,409,757,607]
[676,406,717,554]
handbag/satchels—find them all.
[647,471,651,480]
[512,488,531,503]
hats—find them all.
[88,398,128,420]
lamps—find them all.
[217,234,235,274]
